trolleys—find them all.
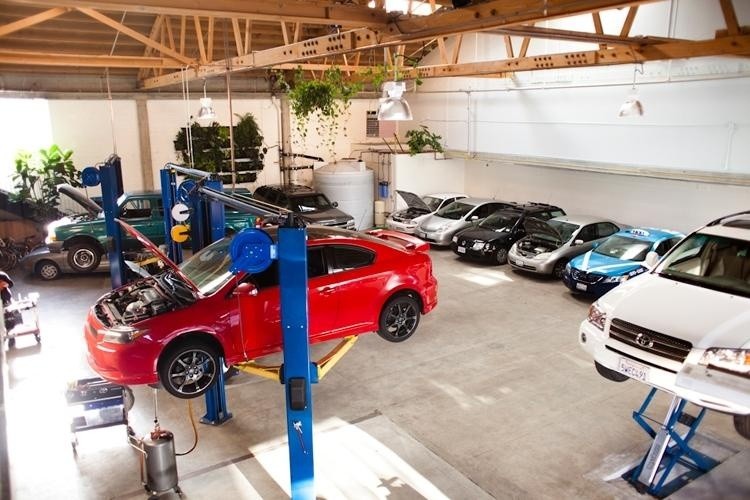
[0,292,41,348]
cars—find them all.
[84,228,438,399]
[21,185,356,279]
[577,210,750,439]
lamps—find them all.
[194,83,217,119]
[376,45,412,121]
[618,63,644,119]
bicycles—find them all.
[1,235,41,273]
